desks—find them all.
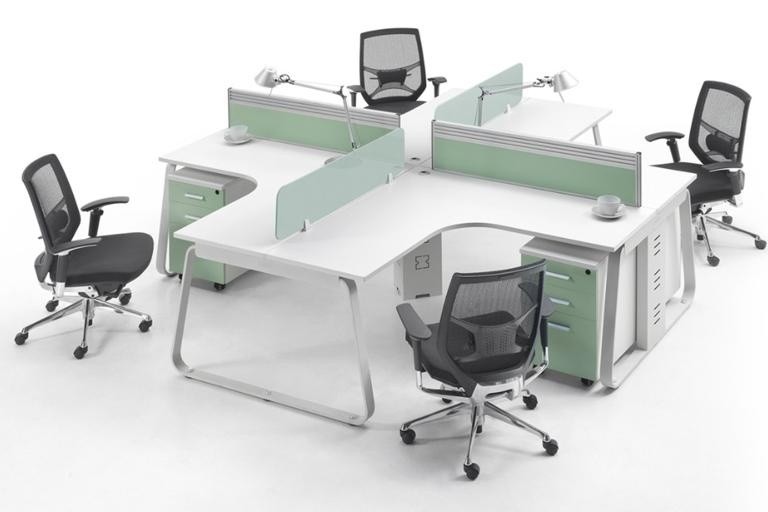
[156,62,699,428]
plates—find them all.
[589,205,626,219]
[223,134,252,146]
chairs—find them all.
[395,257,558,480]
[15,153,153,361]
[346,27,447,115]
[644,81,767,266]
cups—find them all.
[224,125,248,140]
[594,194,625,217]
